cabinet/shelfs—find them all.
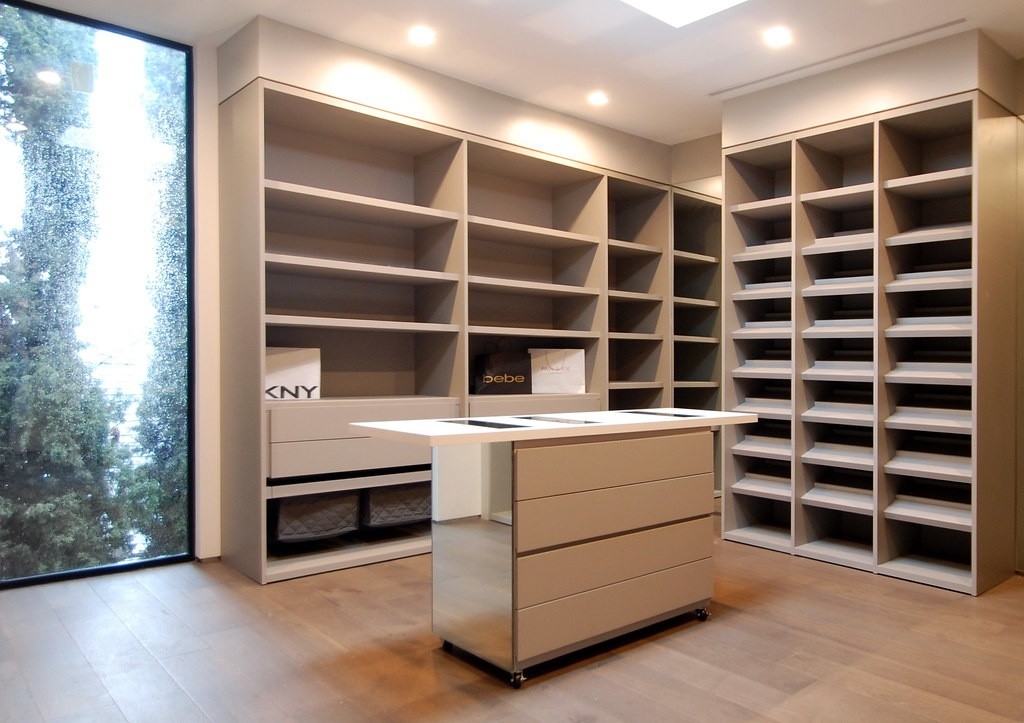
[722,90,1018,598]
[219,76,723,587]
[349,427,758,692]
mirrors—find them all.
[430,440,515,675]
